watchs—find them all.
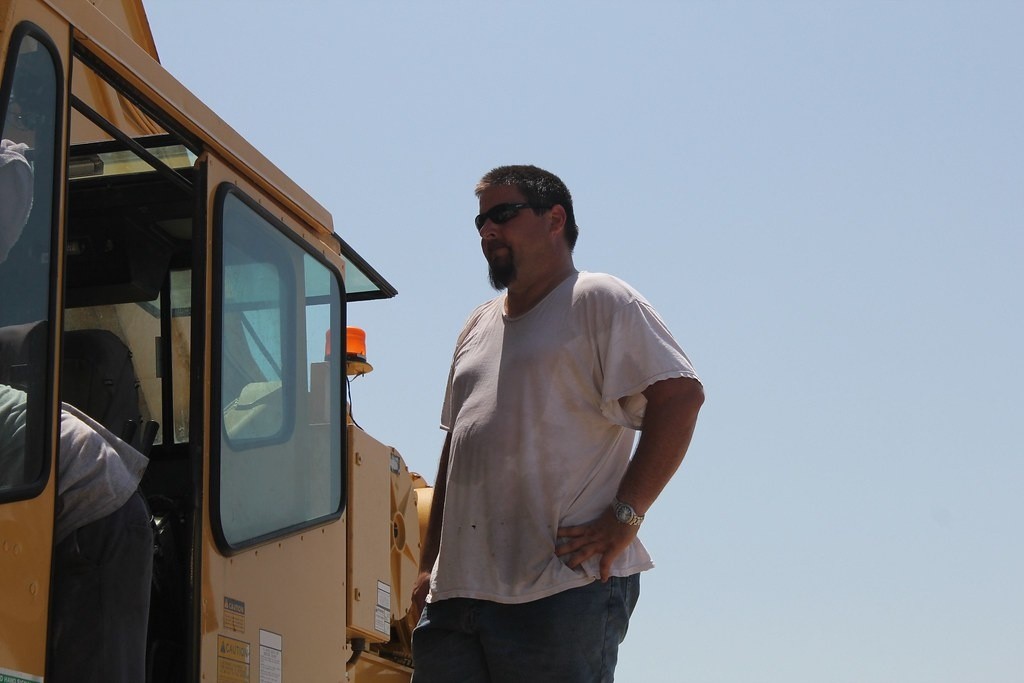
[610,495,645,527]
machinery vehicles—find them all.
[1,2,435,682]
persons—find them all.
[409,164,705,682]
[0,381,156,683]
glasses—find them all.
[475,202,553,231]
